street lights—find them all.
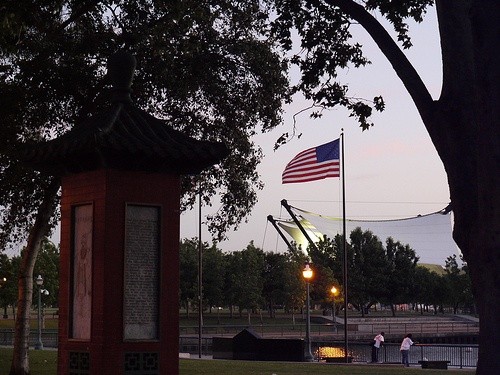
[35,274,44,349]
[330,285,338,332]
[302,264,314,362]
[2,277,8,318]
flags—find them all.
[282,138,340,184]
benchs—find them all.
[417,361,451,369]
[322,356,354,363]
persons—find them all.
[371,332,385,362]
[400,334,417,367]
